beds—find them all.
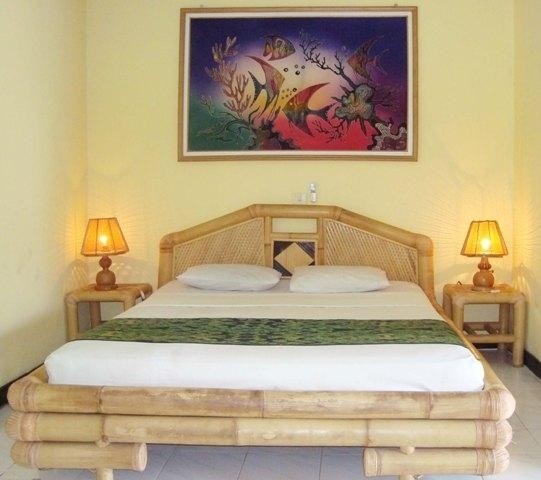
[7,204,516,480]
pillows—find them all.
[290,266,392,294]
[176,263,282,292]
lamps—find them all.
[81,218,129,291]
[460,220,509,291]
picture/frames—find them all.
[178,6,419,162]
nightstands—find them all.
[64,283,153,341]
[442,283,526,367]
